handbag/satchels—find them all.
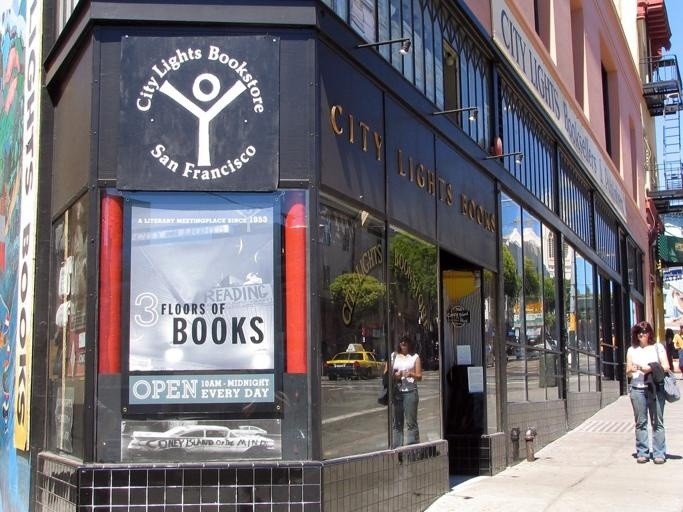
[655,343,681,403]
[377,384,398,406]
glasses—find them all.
[637,328,648,334]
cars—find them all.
[514,336,551,359]
[128,424,275,462]
[324,342,378,381]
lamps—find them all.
[357,38,411,54]
[486,152,523,163]
[432,107,478,121]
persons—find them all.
[381,334,425,450]
[597,324,619,380]
[624,321,672,465]
[666,328,676,371]
[673,323,683,373]
